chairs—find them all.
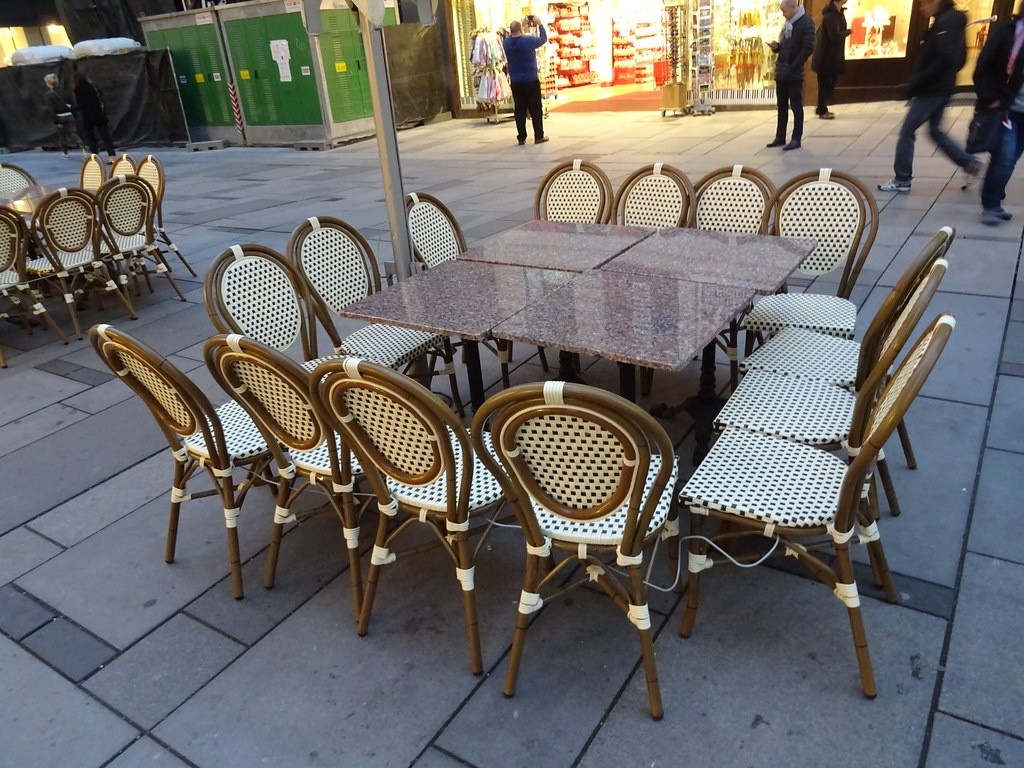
[0,154,956,725]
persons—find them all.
[766,0,816,150]
[811,0,854,119]
[878,0,986,193]
[503,15,550,144]
[45,73,87,160]
[972,1,1024,224]
[66,72,116,165]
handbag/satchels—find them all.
[965,107,1005,153]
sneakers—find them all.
[982,207,1012,222]
[878,180,910,192]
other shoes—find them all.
[819,113,834,120]
[519,140,526,144]
[107,160,114,165]
[767,137,786,147]
[535,136,549,144]
[783,140,801,150]
[61,155,68,160]
[816,109,833,115]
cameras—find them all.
[527,15,534,20]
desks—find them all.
[340,259,576,421]
[598,229,815,399]
[452,214,650,387]
[0,181,98,298]
[493,270,746,423]
